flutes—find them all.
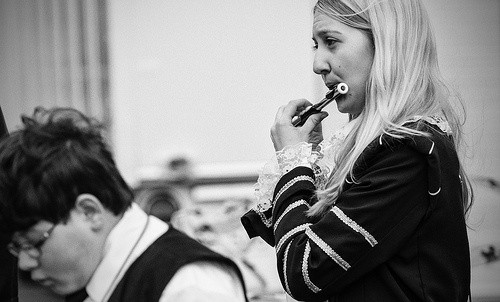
[292,82,349,127]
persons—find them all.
[1,102,251,302]
[237,1,477,302]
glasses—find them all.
[7,218,61,258]
